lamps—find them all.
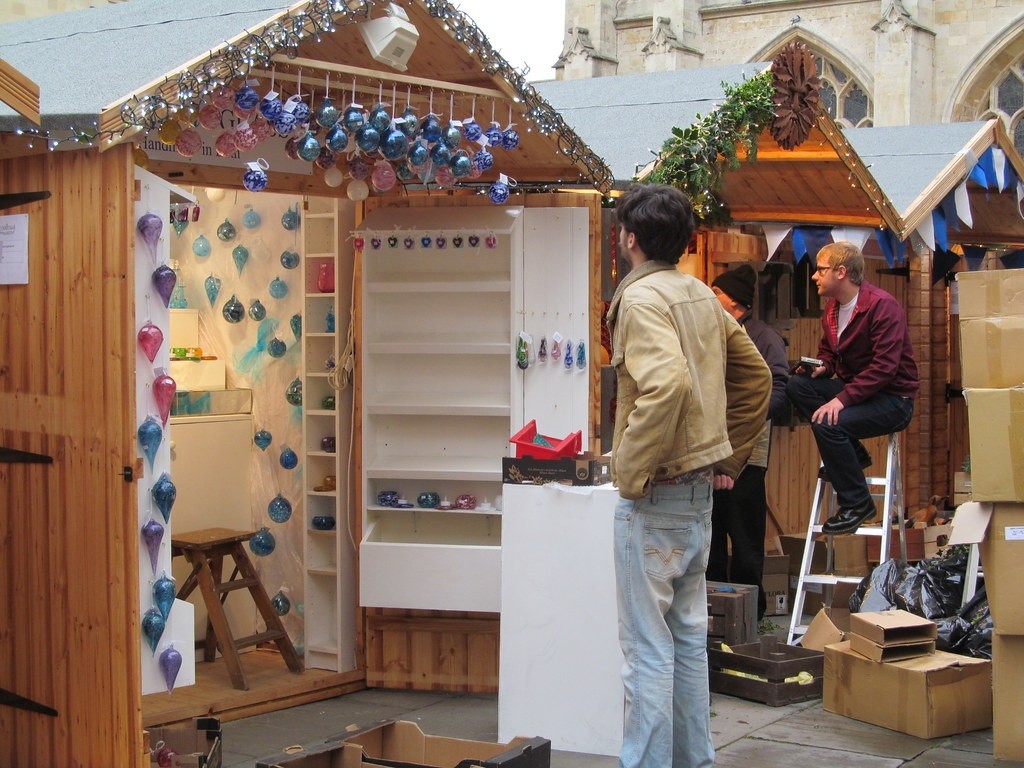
[357,2,420,72]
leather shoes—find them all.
[818,455,872,483]
[822,497,877,535]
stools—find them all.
[170,528,306,691]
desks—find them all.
[498,480,625,756]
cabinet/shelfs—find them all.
[348,205,522,614]
[300,197,362,673]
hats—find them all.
[711,264,757,309]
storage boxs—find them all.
[707,268,1024,760]
[502,450,613,485]
[141,714,224,768]
[256,719,551,768]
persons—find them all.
[786,241,919,534]
[710,264,790,620]
[605,183,773,768]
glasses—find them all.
[815,266,832,271]
